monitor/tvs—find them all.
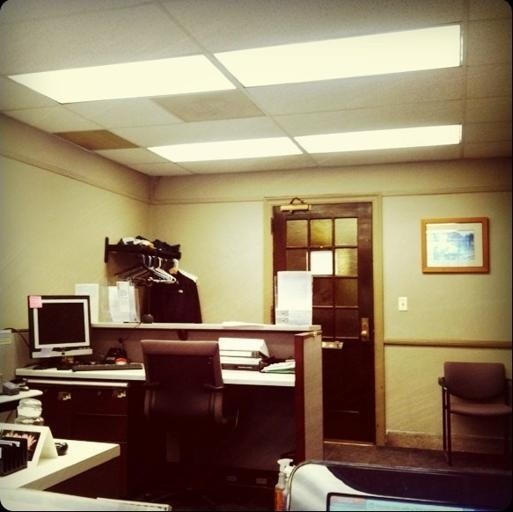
[27,295,94,370]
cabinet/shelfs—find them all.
[22,379,133,499]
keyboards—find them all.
[71,362,143,372]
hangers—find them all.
[114,254,177,285]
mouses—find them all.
[114,358,130,365]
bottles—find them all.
[272,457,294,512]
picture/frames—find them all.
[420,217,489,275]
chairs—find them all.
[437,360,512,466]
[139,337,241,511]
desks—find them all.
[0,438,172,511]
[10,359,296,389]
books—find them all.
[217,335,271,367]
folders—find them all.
[219,338,269,371]
